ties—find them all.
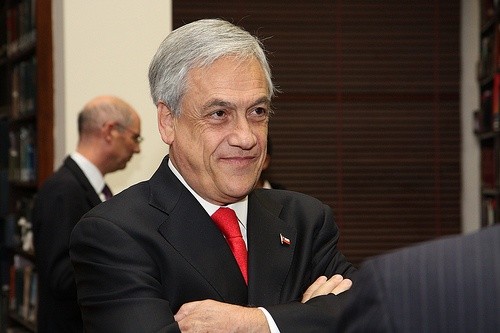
[211,207,248,283]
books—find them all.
[0,0,41,333]
[474,0,500,230]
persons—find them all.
[343,224,500,333]
[69,19,355,333]
[33,96,143,333]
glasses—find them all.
[103,121,144,147]
